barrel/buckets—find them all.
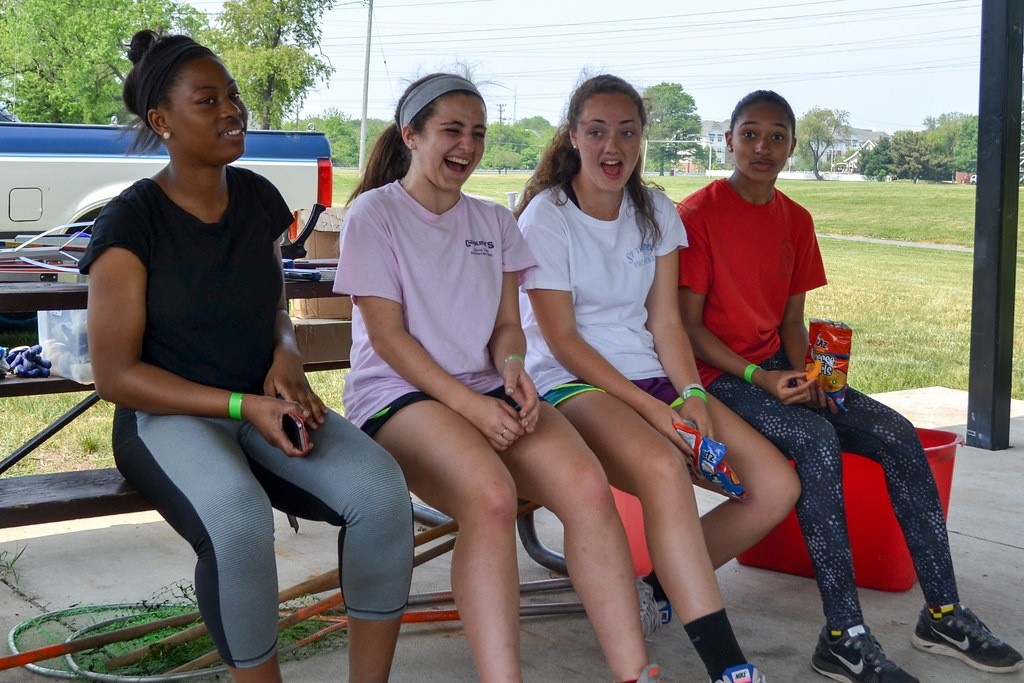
[608,486,654,575]
[736,426,964,592]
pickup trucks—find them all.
[1,119,333,254]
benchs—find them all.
[0,363,350,399]
[0,467,569,579]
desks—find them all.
[0,271,350,475]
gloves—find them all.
[5,345,52,378]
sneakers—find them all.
[809,625,920,683]
[635,575,671,639]
[911,602,1024,673]
[716,663,767,683]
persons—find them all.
[77,30,414,683]
[676,90,1023,683]
[514,75,802,682]
[332,73,670,683]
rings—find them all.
[500,427,507,433]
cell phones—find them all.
[282,413,308,452]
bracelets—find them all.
[504,354,523,363]
[229,392,244,420]
[683,383,708,404]
[744,364,760,384]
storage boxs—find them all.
[37,307,92,385]
[288,207,353,362]
[733,428,965,592]
